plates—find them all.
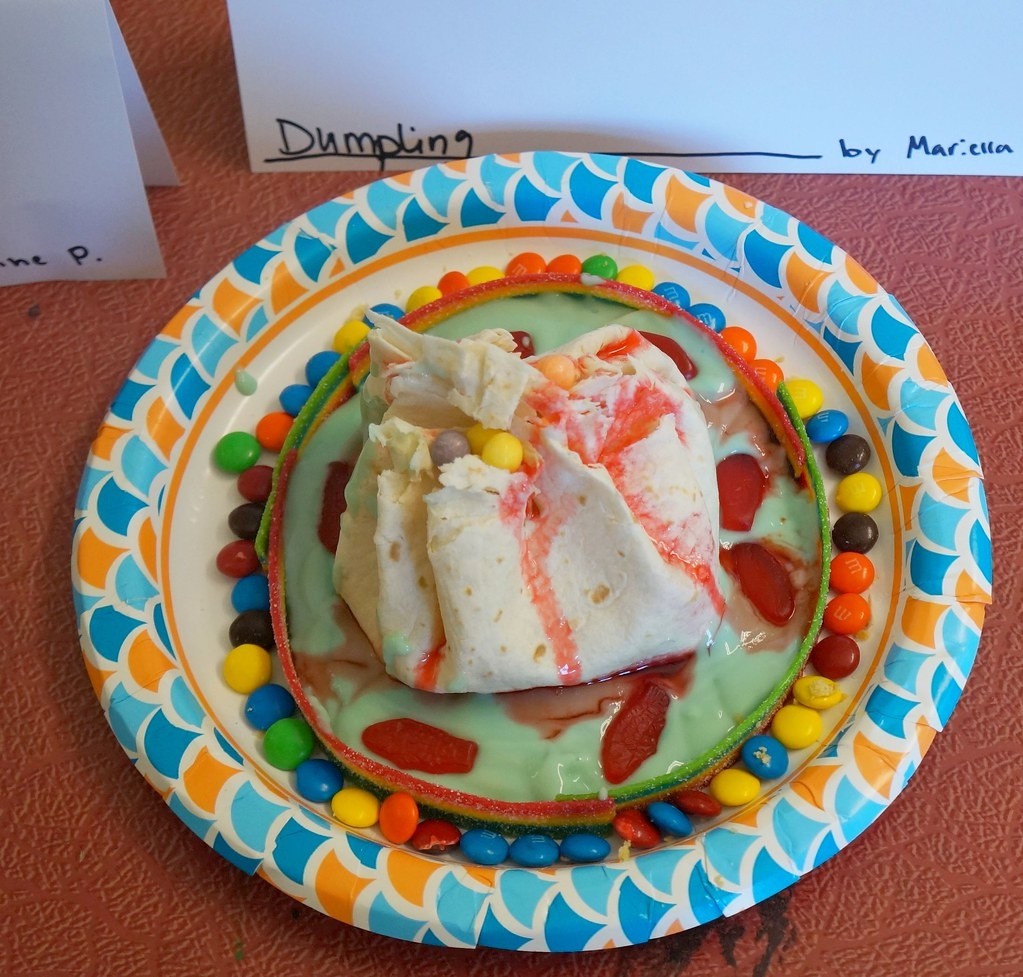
[72,149,994,952]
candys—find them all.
[209,252,881,871]
